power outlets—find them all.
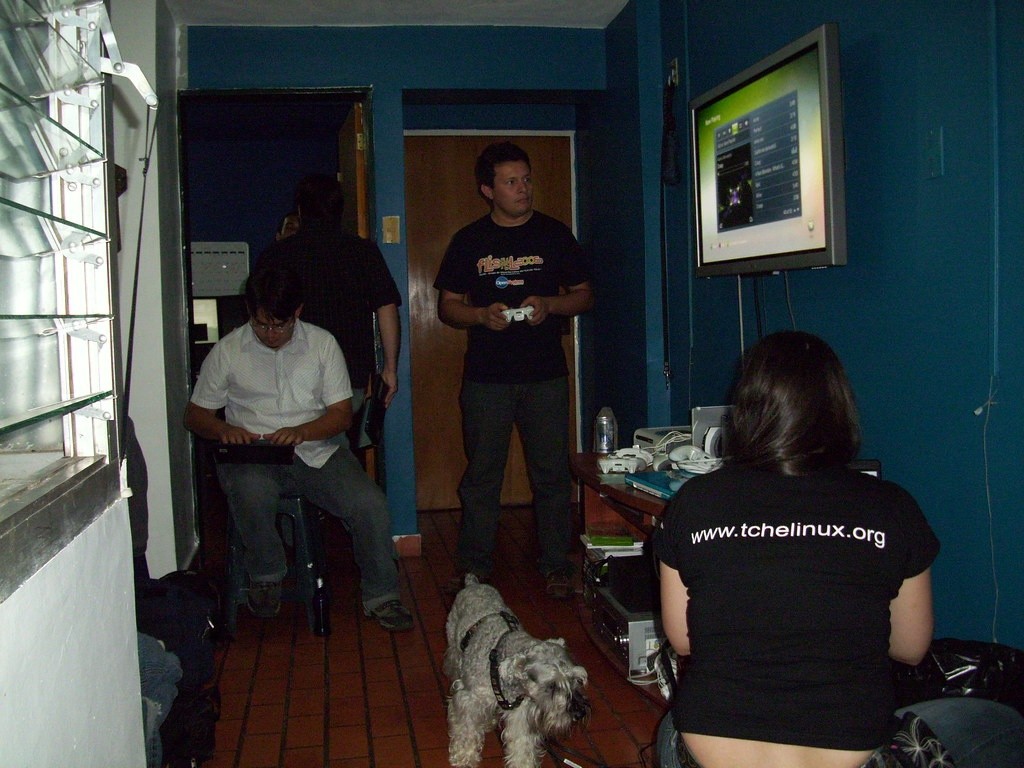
[921,125,944,180]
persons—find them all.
[183,272,414,632]
[244,170,402,603]
[651,329,932,768]
[276,212,300,241]
[432,141,599,600]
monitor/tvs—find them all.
[687,23,847,279]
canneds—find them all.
[595,416,614,453]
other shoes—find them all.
[444,568,487,595]
[547,571,574,599]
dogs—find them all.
[444,572,590,768]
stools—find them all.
[222,492,331,636]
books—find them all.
[580,534,644,566]
[628,618,668,679]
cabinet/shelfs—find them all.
[566,452,672,712]
[0,0,148,768]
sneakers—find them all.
[250,580,281,618]
[372,599,414,631]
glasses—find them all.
[251,311,295,333]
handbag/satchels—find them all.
[136,580,219,695]
